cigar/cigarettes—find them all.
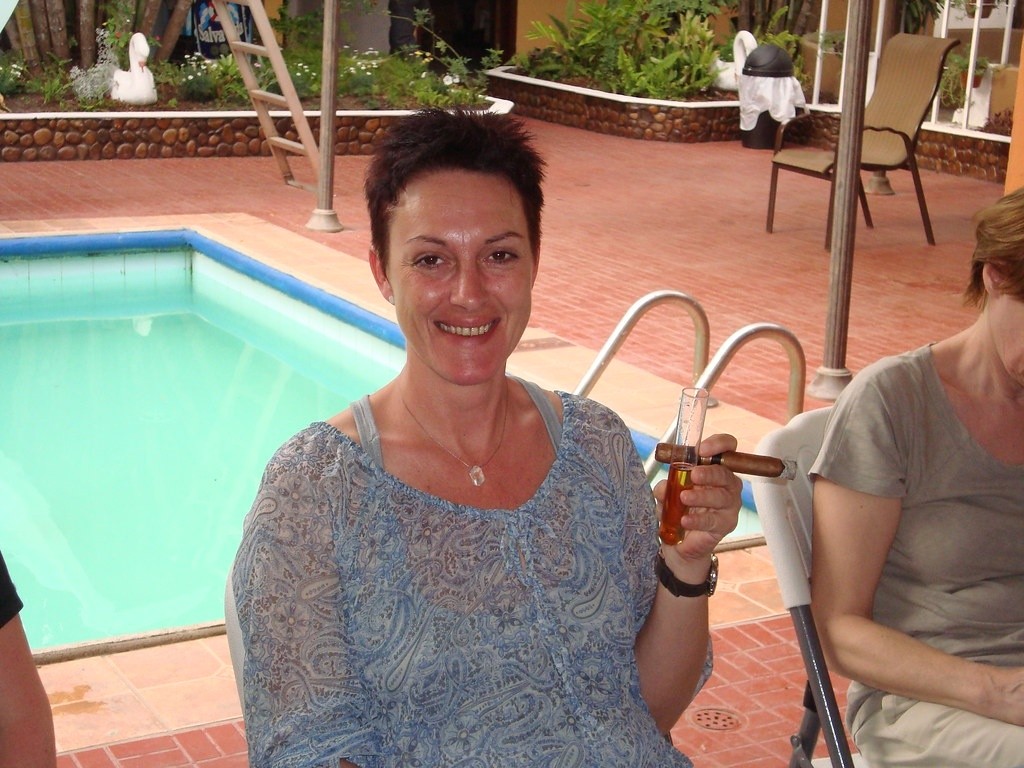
[654,443,798,481]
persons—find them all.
[808,186,1024,768]
[223,107,744,768]
[0,551,59,768]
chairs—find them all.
[765,31,961,253]
[751,405,860,768]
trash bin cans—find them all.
[741,44,794,150]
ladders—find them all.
[214,2,321,193]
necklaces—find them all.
[395,375,509,488]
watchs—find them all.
[654,545,719,598]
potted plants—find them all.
[798,27,846,93]
[940,0,1004,22]
[937,43,1015,110]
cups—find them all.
[660,388,709,546]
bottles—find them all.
[192,0,246,60]
